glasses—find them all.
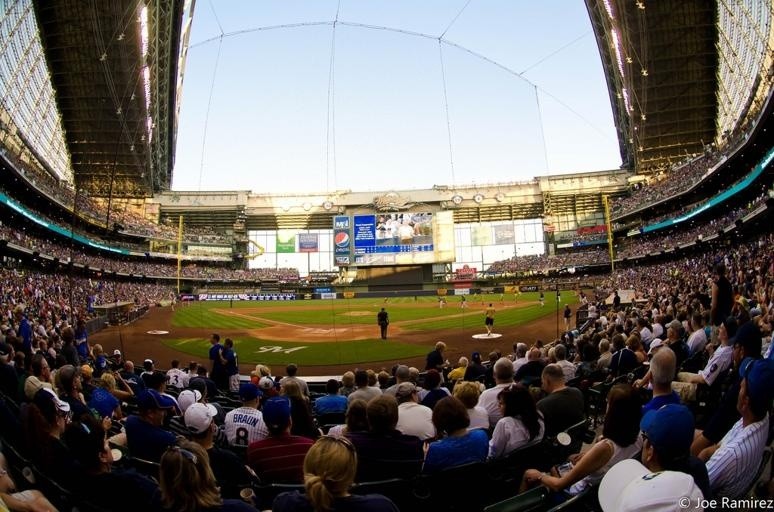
[320,433,353,451]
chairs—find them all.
[100,369,608,511]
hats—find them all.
[640,403,695,461]
[26,360,291,433]
[598,459,704,511]
[739,357,774,401]
[395,366,410,384]
[11,305,24,315]
[646,318,680,355]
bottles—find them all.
[586,405,595,429]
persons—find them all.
[0,86,774,512]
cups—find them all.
[643,362,649,368]
[627,372,633,382]
[108,449,124,470]
[556,432,571,456]
[239,488,256,505]
[22,466,36,483]
